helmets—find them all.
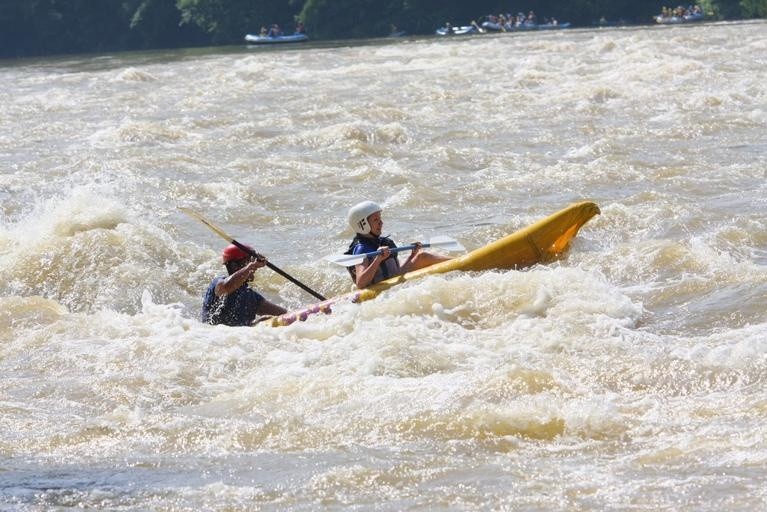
[347,201,383,235]
[221,243,256,265]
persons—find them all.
[600,15,608,23]
[551,16,558,24]
[662,4,702,16]
[342,199,452,290]
[259,23,268,35]
[269,22,281,36]
[488,8,536,28]
[200,245,287,328]
[544,16,551,24]
[469,19,478,31]
[445,21,452,31]
[295,22,305,33]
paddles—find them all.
[324,234,465,267]
[182,208,326,300]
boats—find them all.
[242,31,309,44]
[652,12,708,25]
[259,198,610,331]
[431,18,574,37]
